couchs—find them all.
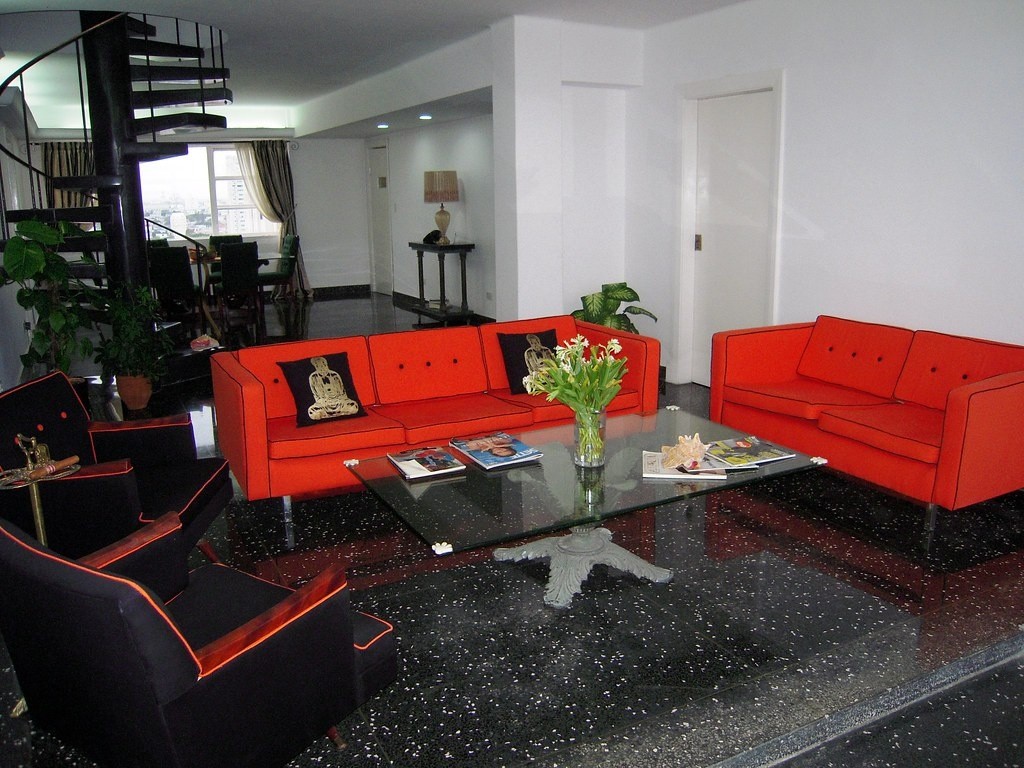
[0,507,398,766]
[709,312,1024,548]
[208,309,663,552]
[0,367,233,565]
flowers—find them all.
[527,327,629,419]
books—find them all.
[450,430,544,471]
[385,446,467,480]
[684,454,759,473]
[702,435,797,467]
[643,450,727,480]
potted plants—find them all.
[3,217,99,405]
[103,280,172,417]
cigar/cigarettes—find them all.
[28,455,79,480]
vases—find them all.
[572,407,607,471]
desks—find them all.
[0,461,83,551]
[408,239,475,326]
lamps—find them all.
[422,168,460,245]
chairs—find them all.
[206,233,301,340]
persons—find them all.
[464,432,517,457]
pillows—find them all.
[274,352,369,428]
[494,329,559,394]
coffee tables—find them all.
[342,404,827,610]
